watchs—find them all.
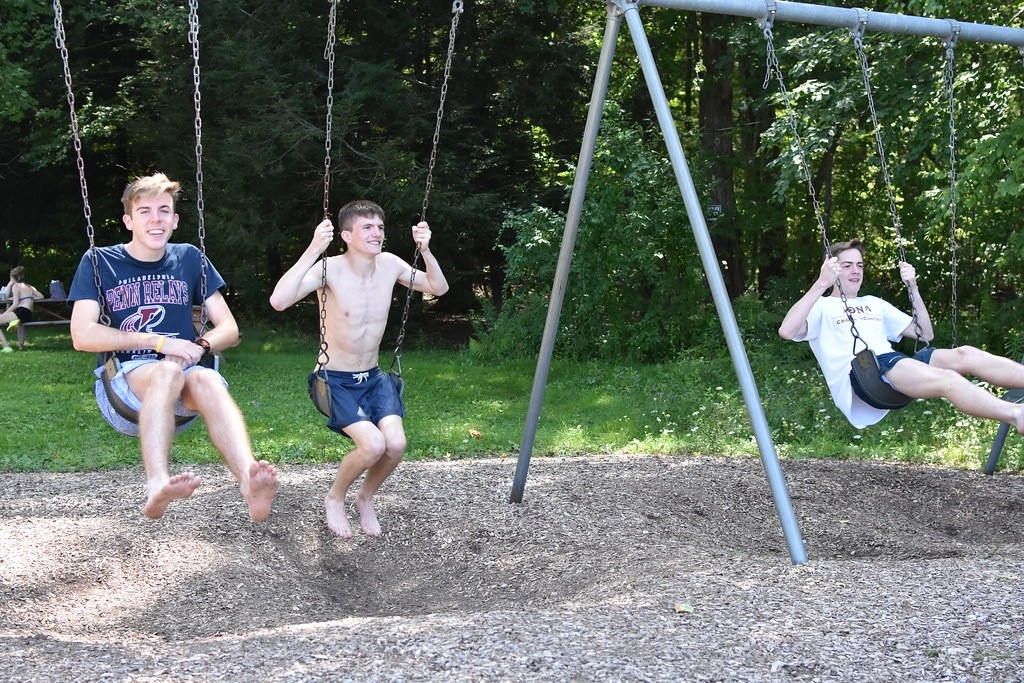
[193,339,210,358]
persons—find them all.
[779,238,1024,437]
[0,266,44,353]
[269,200,449,539]
[67,173,279,521]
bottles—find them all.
[1,286,7,301]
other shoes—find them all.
[0,347,13,353]
[7,319,21,333]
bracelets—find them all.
[156,335,166,353]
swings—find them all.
[944,47,1024,403]
[762,16,930,407]
[51,0,222,426]
[310,0,467,418]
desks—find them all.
[0,299,72,321]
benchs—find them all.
[15,320,71,350]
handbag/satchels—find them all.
[50,280,66,299]
